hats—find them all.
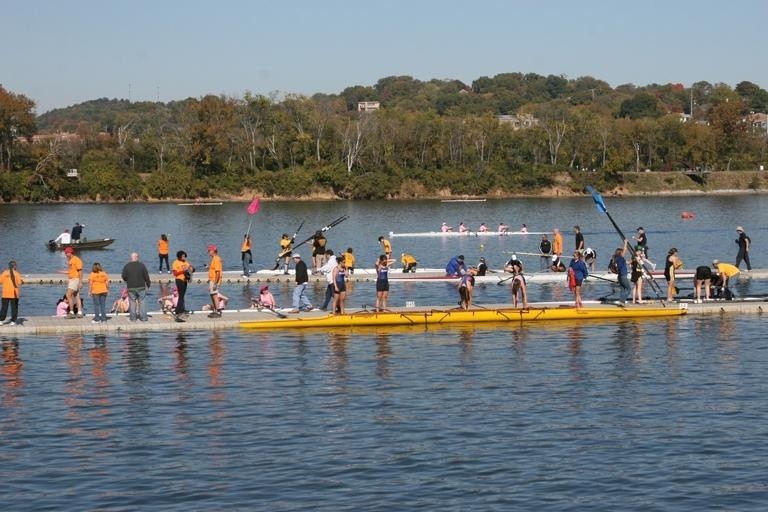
[259,285,269,291]
[292,253,301,259]
[552,255,557,261]
[64,246,75,254]
[736,227,744,232]
[512,255,516,260]
[208,245,218,252]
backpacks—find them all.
[608,256,620,274]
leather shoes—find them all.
[287,308,300,314]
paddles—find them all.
[674,286,723,294]
[279,215,349,257]
[252,298,286,319]
[498,273,523,284]
[167,307,186,322]
[271,219,304,270]
[587,187,665,307]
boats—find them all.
[232,304,691,331]
[372,267,725,285]
[49,304,322,315]
[254,268,521,274]
[440,198,488,203]
[529,292,768,303]
[46,236,119,254]
[177,202,224,206]
[388,230,558,238]
[242,315,686,333]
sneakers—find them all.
[208,312,221,318]
[64,312,76,319]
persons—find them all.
[64,247,84,319]
[441,222,527,233]
[56,295,70,316]
[503,255,528,309]
[735,226,751,270]
[377,237,417,312]
[444,255,487,309]
[539,223,597,308]
[156,230,355,316]
[0,261,22,325]
[72,222,82,244]
[88,263,109,323]
[121,252,151,321]
[55,229,71,244]
[111,288,130,313]
[664,248,680,302]
[693,260,741,299]
[608,227,648,307]
